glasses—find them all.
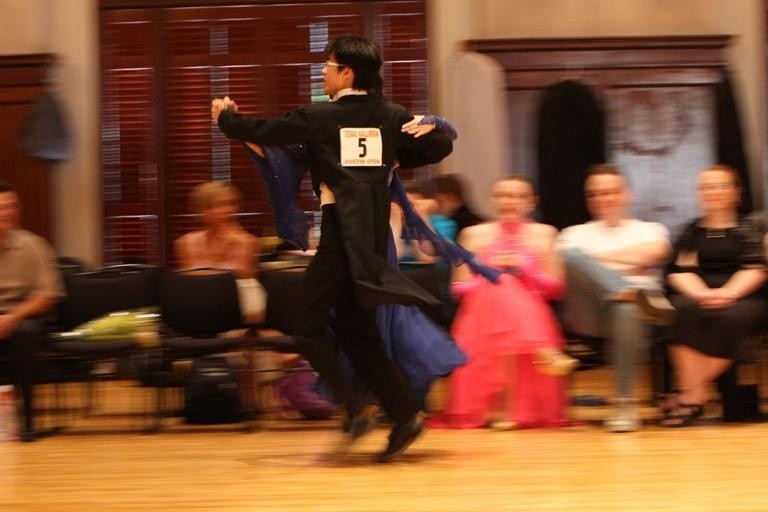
[327,60,345,68]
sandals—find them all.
[651,389,702,426]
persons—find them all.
[431,174,471,231]
[555,167,670,432]
[0,184,67,375]
[167,181,264,421]
[447,178,565,431]
[654,167,765,427]
[210,36,456,460]
[219,78,468,433]
[384,186,453,272]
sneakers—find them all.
[535,347,579,376]
[604,396,639,432]
[341,403,378,438]
[370,409,433,461]
